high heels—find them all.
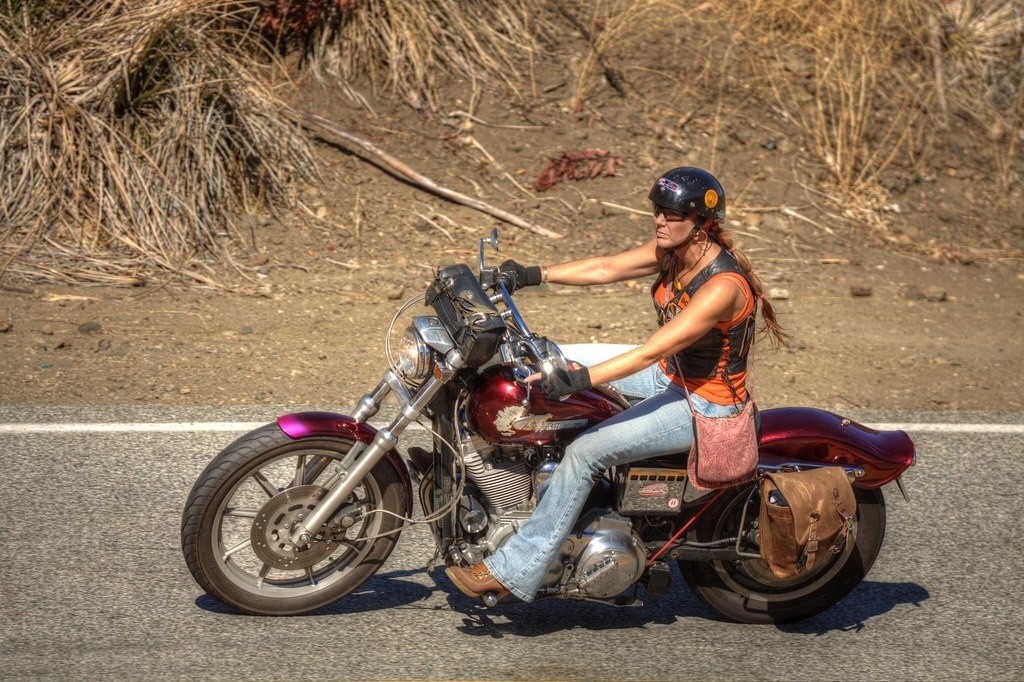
[444,562,512,601]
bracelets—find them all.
[543,266,548,283]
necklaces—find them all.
[675,230,712,290]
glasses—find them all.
[652,202,691,222]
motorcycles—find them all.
[181,230,917,626]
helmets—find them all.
[648,167,726,224]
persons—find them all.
[444,166,794,601]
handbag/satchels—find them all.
[687,401,758,491]
[758,466,857,581]
